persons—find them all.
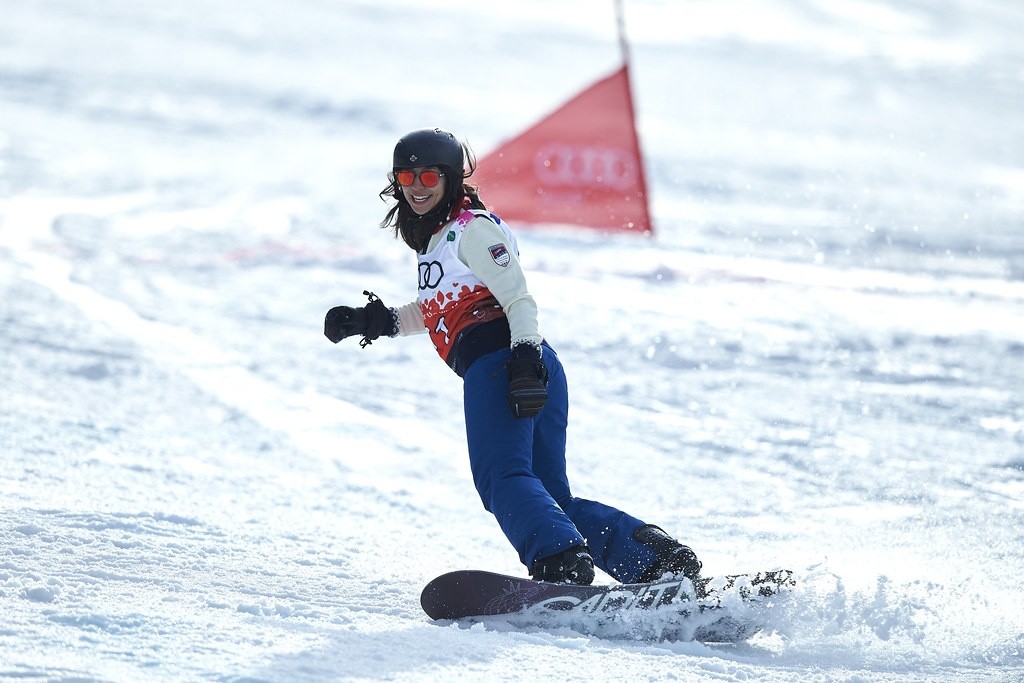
[324,128,702,581]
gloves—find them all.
[324,290,394,349]
[492,341,550,419]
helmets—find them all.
[393,128,464,176]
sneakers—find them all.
[529,545,595,586]
[633,524,703,583]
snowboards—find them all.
[419,566,796,644]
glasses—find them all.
[394,169,445,187]
